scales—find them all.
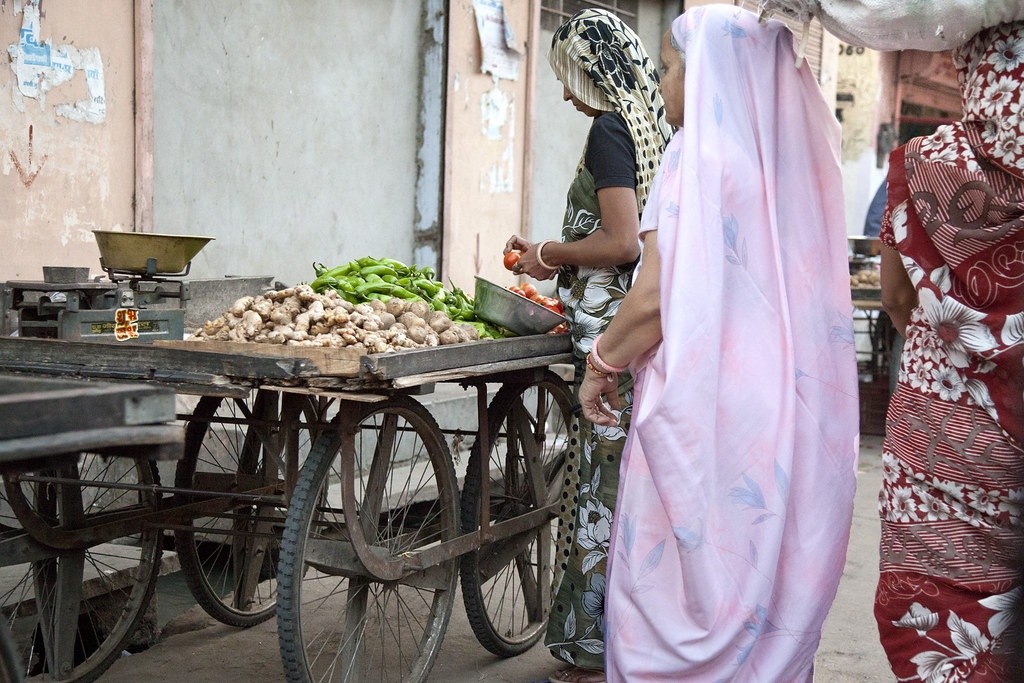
[4,229,216,346]
[847,236,882,272]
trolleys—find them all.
[1,276,594,683]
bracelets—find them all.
[536,239,563,270]
[592,333,631,372]
[586,353,614,382]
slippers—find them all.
[548,664,606,683]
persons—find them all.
[503,9,679,683]
[578,3,859,683]
[874,20,1024,683]
[863,173,906,396]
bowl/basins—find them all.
[91,230,216,274]
[472,274,569,337]
[847,236,883,256]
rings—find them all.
[513,261,522,271]
